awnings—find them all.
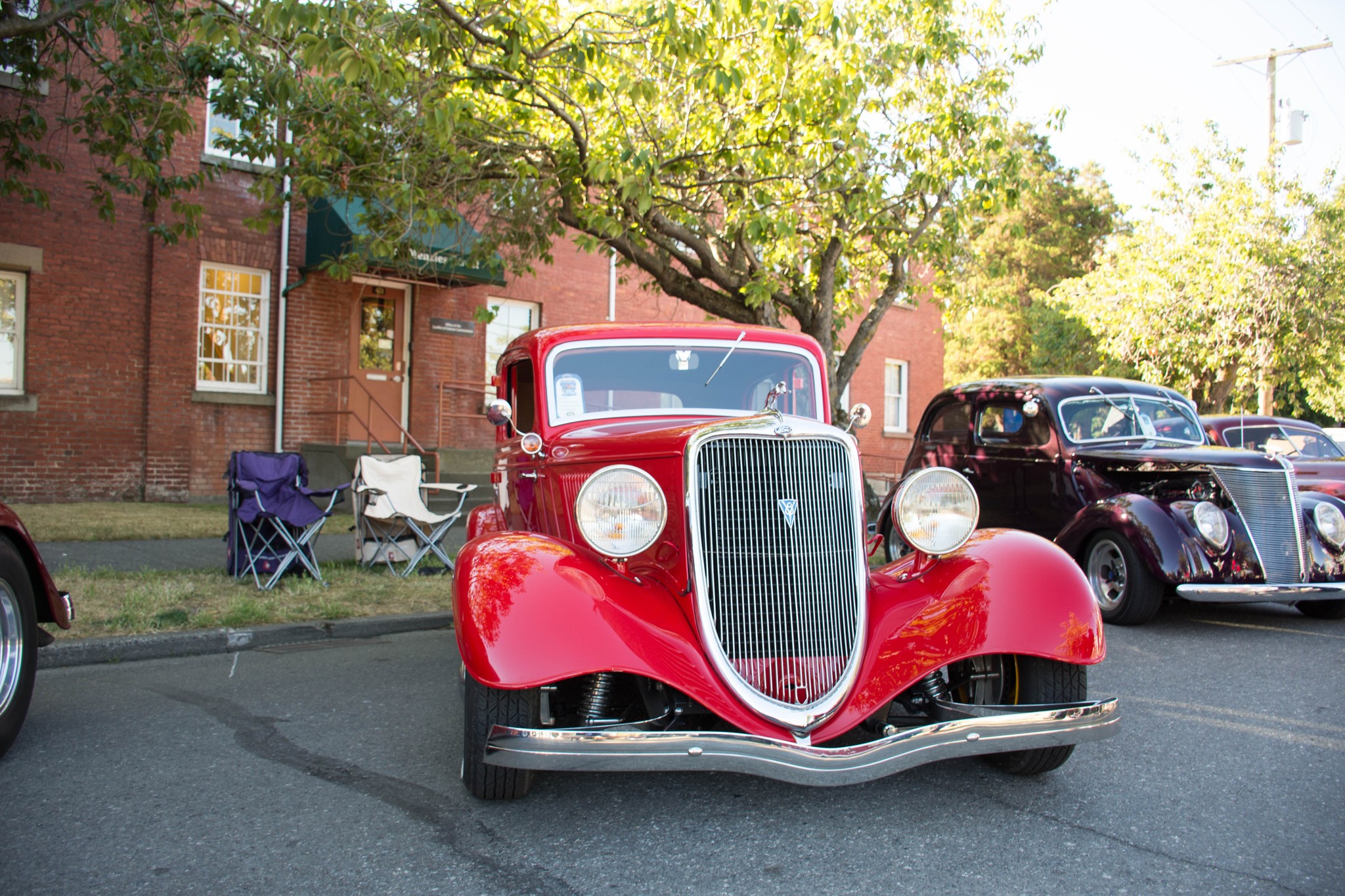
[302,182,509,290]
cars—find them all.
[869,373,1345,628]
[0,499,79,756]
[452,322,1121,801]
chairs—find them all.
[223,450,480,590]
